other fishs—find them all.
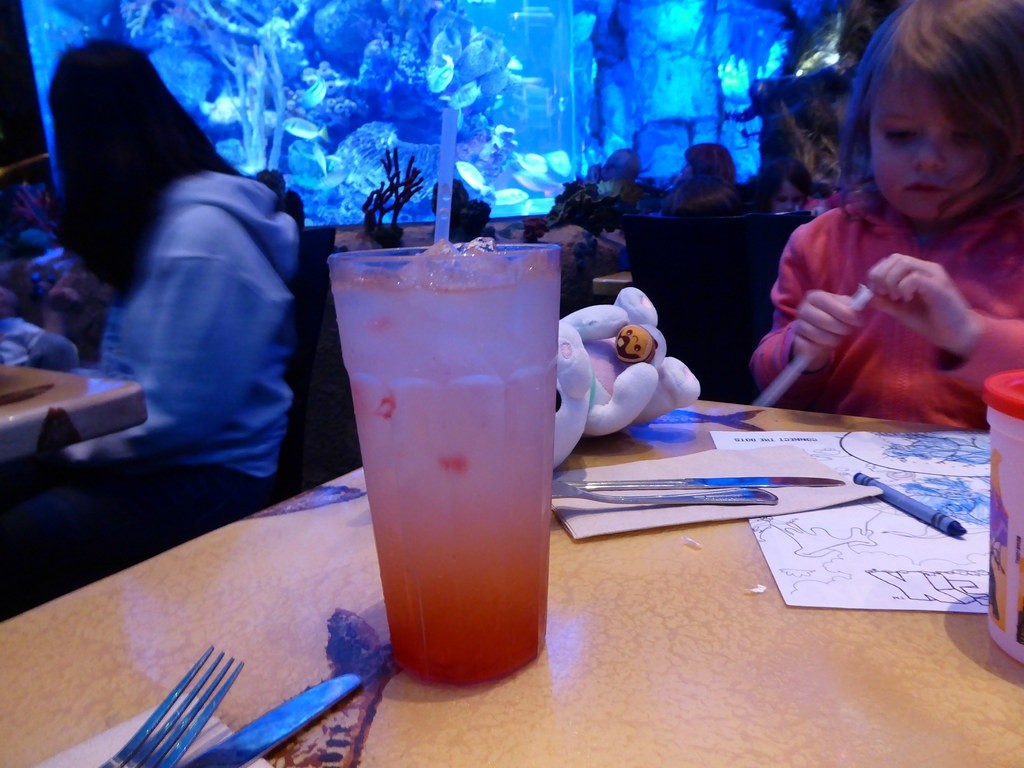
[272,21,589,206]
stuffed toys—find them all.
[552,285,702,473]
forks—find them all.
[100,646,243,768]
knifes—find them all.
[558,476,846,491]
[182,673,363,768]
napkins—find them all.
[36,694,274,768]
[551,447,884,539]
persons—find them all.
[430,142,812,245]
[0,287,78,373]
[0,36,305,618]
[748,0,1024,430]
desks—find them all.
[0,367,1024,768]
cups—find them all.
[327,244,561,687]
[981,368,1024,664]
